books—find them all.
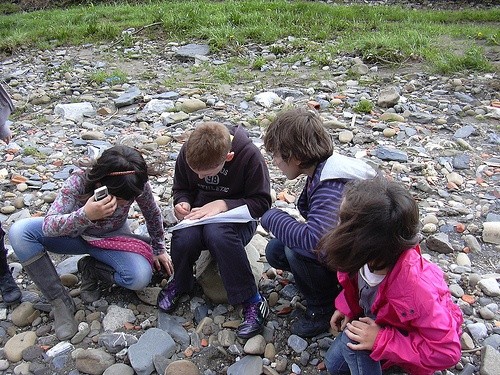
[169,204,261,232]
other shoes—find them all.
[294,309,336,337]
[0,268,22,304]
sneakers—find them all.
[236,295,270,338]
[158,275,186,311]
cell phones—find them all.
[94,185,108,201]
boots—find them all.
[77,256,117,305]
[22,250,77,341]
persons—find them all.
[156,119,272,340]
[0,221,24,308]
[258,108,383,338]
[6,145,176,341]
[317,175,465,375]
